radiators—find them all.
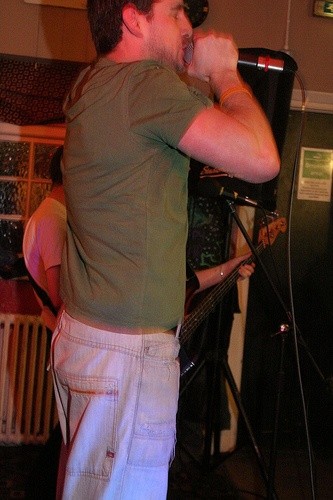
[0,314,58,445]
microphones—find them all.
[204,180,263,209]
[183,41,295,78]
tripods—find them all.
[178,200,278,500]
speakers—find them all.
[192,47,297,209]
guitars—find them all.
[179,209,288,347]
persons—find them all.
[23,144,257,338]
[50,0,281,500]
